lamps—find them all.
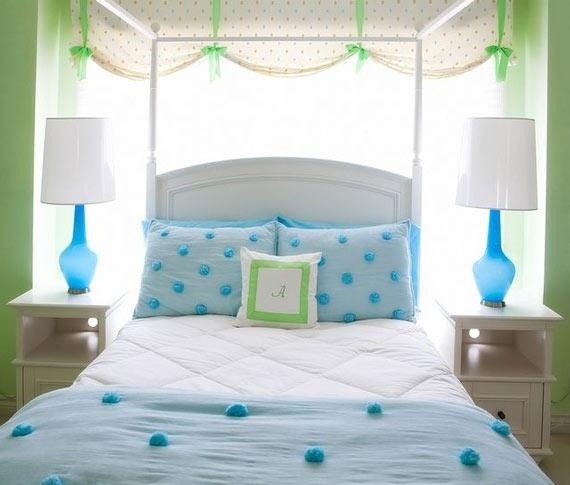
[40,118,117,294]
[455,117,537,308]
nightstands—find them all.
[6,275,128,411]
[433,300,563,465]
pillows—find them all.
[234,246,322,328]
[277,215,419,307]
[131,220,275,318]
[136,218,275,237]
[276,223,415,321]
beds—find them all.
[1,157,555,484]
